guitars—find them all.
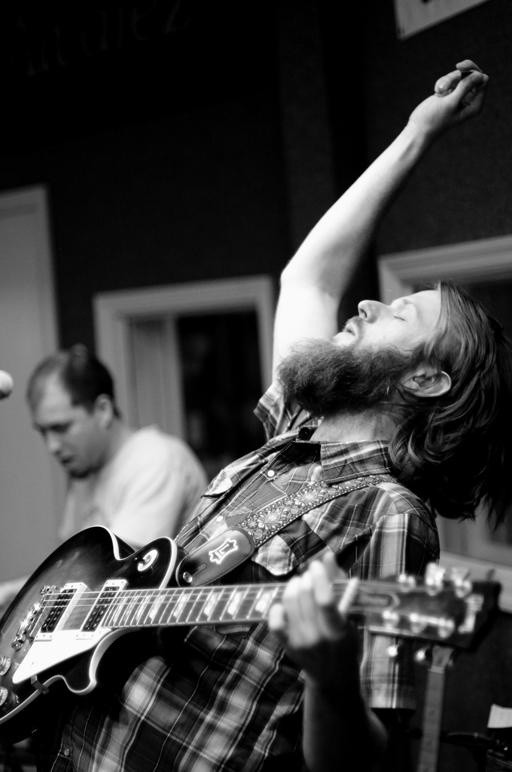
[0,526,486,746]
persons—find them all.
[23,343,209,555]
[41,59,511,771]
